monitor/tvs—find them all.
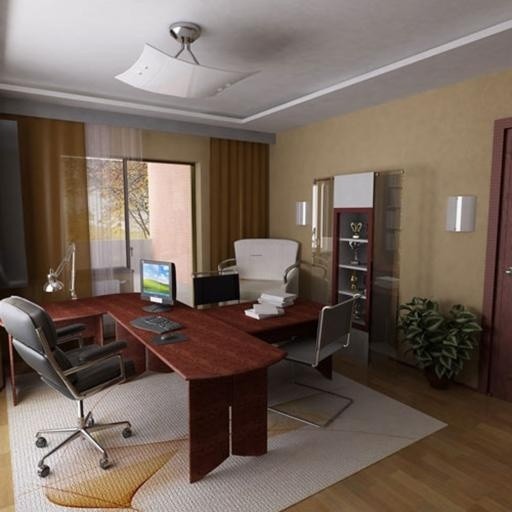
[139,258,177,313]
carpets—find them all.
[4,373,451,510]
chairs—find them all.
[191,236,301,309]
[267,293,363,432]
[0,293,136,478]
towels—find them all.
[245,287,298,320]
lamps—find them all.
[43,240,78,302]
[113,22,263,101]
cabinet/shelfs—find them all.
[331,208,375,331]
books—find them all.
[244,293,297,320]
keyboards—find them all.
[128,314,183,335]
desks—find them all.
[200,298,335,381]
[0,290,290,485]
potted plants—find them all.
[397,293,484,392]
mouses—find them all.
[161,332,176,340]
